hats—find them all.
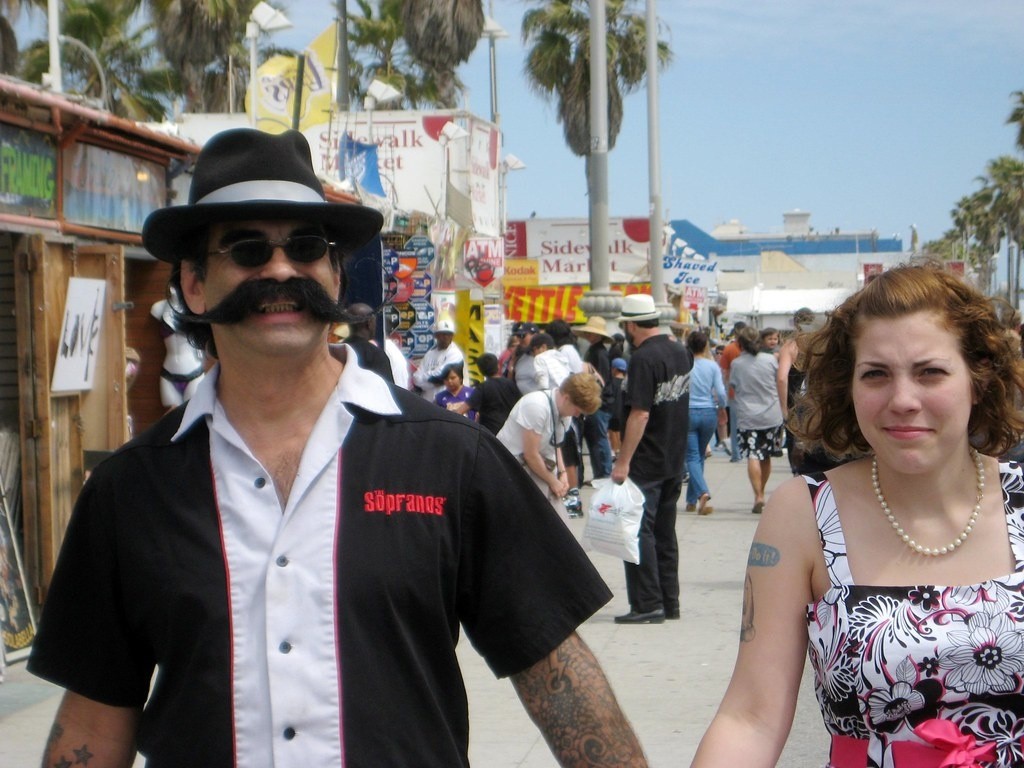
[511,322,540,335]
[615,294,661,321]
[612,358,628,371]
[142,129,383,264]
[434,320,454,335]
[570,316,615,344]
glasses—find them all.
[204,235,335,268]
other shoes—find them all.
[564,488,583,516]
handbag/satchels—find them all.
[580,476,646,565]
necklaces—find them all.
[871,446,984,556]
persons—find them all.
[612,294,690,626]
[151,283,205,405]
[691,267,1024,768]
[27,130,649,768]
[412,319,468,404]
[453,353,522,438]
[333,303,408,390]
[495,317,629,501]
[683,307,816,514]
[1000,309,1021,351]
[431,363,479,424]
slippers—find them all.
[698,507,712,516]
[686,504,696,512]
[752,501,764,514]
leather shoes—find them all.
[615,606,680,624]
